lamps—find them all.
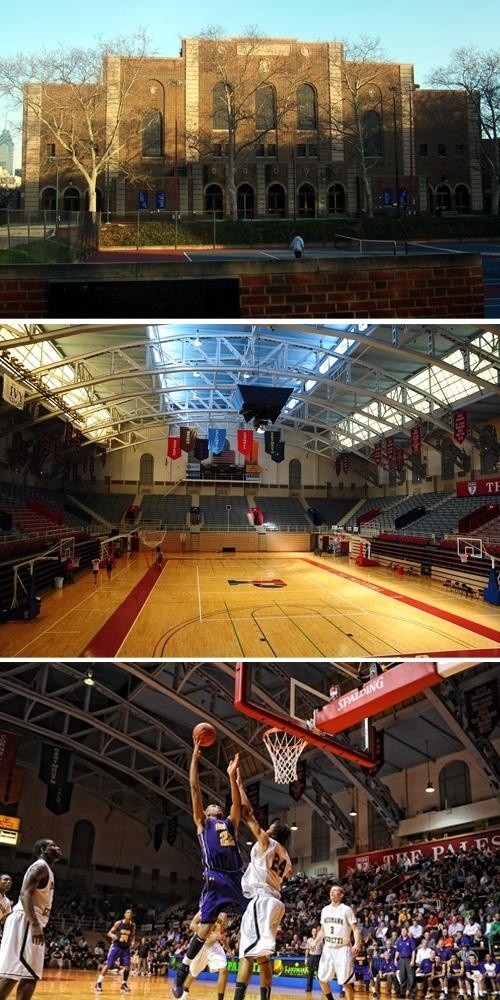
[425,738,435,793]
[289,800,298,831]
[348,783,358,817]
[82,666,95,686]
[245,830,253,846]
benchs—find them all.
[0,482,500,556]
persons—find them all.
[1,870,199,978]
[0,839,63,1000]
[95,909,137,993]
[128,551,136,560]
[181,895,234,999]
[213,837,500,1000]
[173,735,254,999]
[235,767,293,1000]
[290,232,305,257]
[105,557,113,581]
[155,551,164,572]
[92,558,100,583]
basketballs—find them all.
[193,723,216,747]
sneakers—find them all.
[96,983,102,990]
[121,983,130,992]
[172,975,182,998]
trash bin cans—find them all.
[54,577,64,589]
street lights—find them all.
[388,84,401,221]
[408,82,420,176]
